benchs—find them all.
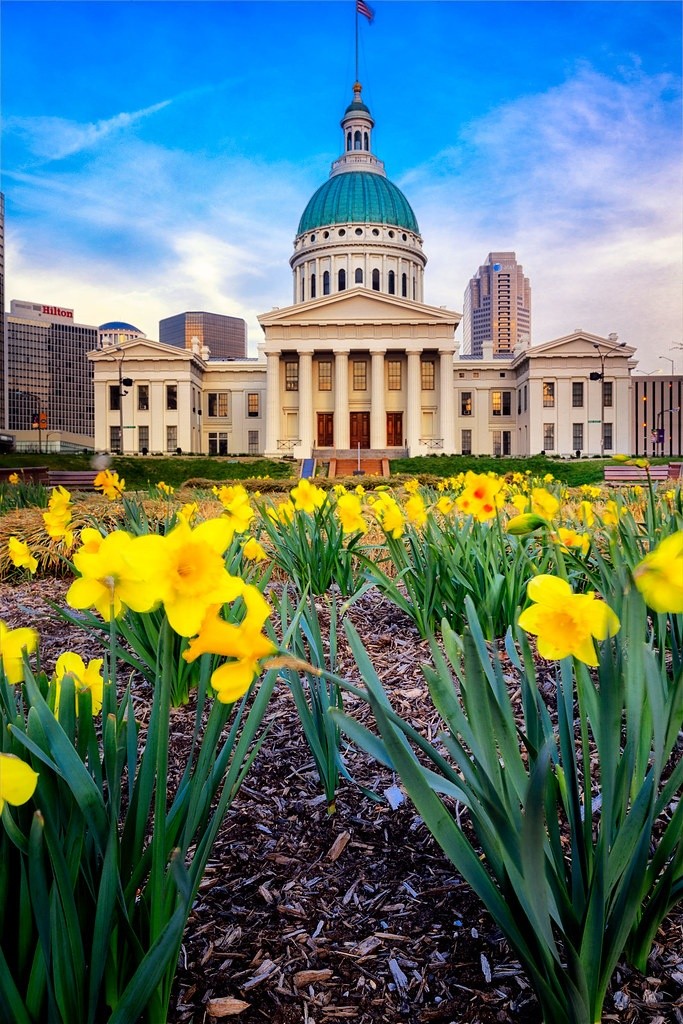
[0,466,117,492]
[604,462,683,488]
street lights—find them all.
[21,392,42,453]
[593,342,628,459]
[658,355,674,375]
[651,407,679,456]
[94,346,133,454]
[46,431,62,454]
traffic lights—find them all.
[31,413,39,429]
[652,429,658,442]
